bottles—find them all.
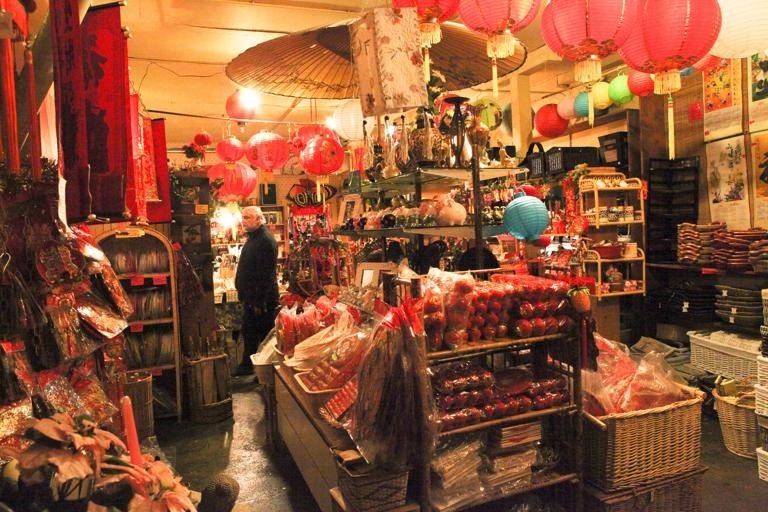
[599,205,642,223]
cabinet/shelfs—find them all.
[82,168,683,512]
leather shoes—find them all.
[230,367,253,377]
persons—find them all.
[232,206,277,377]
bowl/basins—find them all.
[593,245,622,258]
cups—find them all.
[620,242,638,258]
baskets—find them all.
[329,441,411,511]
[581,381,702,494]
[319,403,352,430]
[577,461,709,511]
[688,326,768,482]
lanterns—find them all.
[505,192,550,243]
[417,262,597,433]
